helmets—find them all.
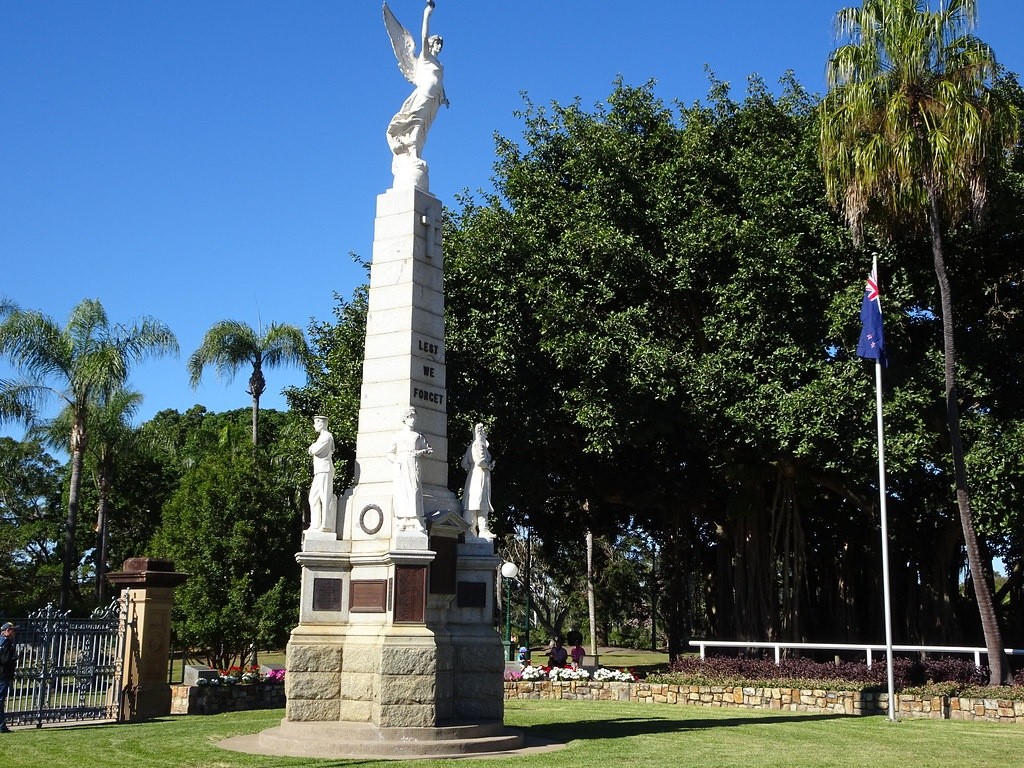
[519,647,528,652]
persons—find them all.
[570,640,585,669]
[300,415,335,535]
[382,1,450,157]
[460,423,498,539]
[517,646,531,671]
[385,406,435,532]
[546,638,568,668]
[0,621,18,733]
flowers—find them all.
[195,663,286,686]
[505,667,638,686]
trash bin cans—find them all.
[516,646,531,666]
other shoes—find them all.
[0,728,11,733]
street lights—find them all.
[501,561,518,660]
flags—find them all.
[855,264,888,371]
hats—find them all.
[1,622,13,630]
